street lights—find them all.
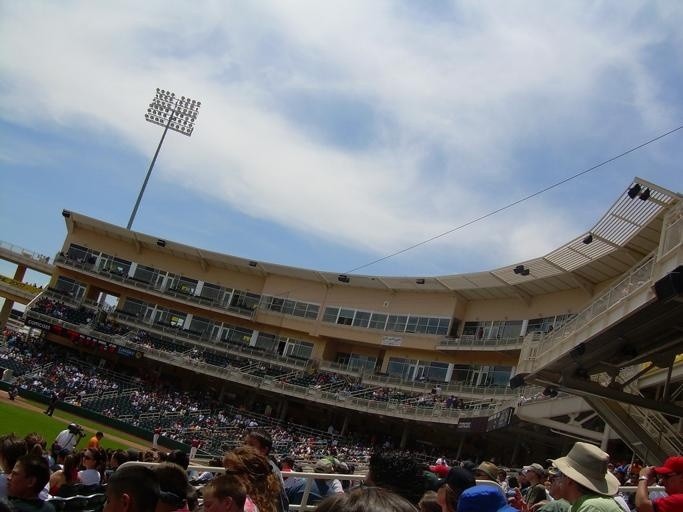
[126,88,201,232]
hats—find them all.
[655,454,683,474]
[551,440,620,498]
[446,467,476,491]
[429,464,449,475]
[472,460,499,481]
[456,484,517,511]
[524,462,545,477]
[314,459,334,473]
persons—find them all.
[251,359,682,511]
[1,249,252,511]
